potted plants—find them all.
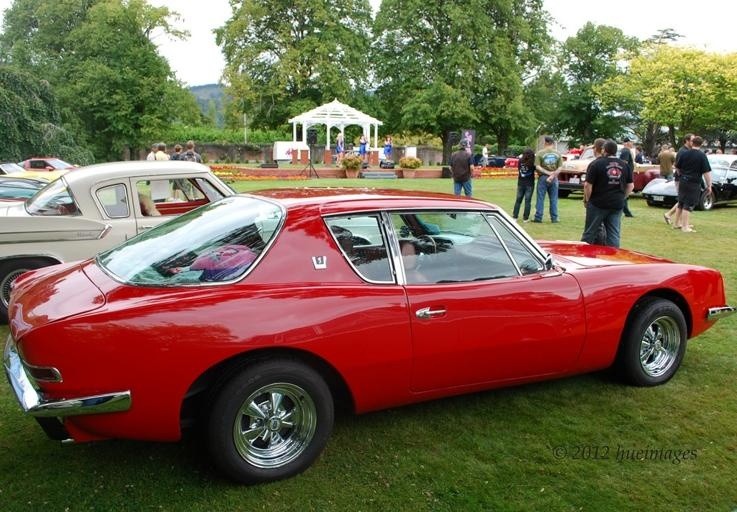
[340,153,365,179]
[399,155,421,178]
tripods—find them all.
[299,145,319,179]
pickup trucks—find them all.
[549,144,661,200]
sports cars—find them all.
[640,154,737,211]
[0,188,736,484]
[0,158,240,317]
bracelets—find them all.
[582,200,588,203]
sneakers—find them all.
[664,213,696,233]
[513,215,559,223]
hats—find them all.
[624,136,635,142]
[460,139,467,146]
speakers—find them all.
[306,129,318,145]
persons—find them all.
[383,135,392,160]
[634,142,736,166]
[662,133,695,228]
[178,140,203,200]
[463,132,471,142]
[582,138,607,246]
[169,144,183,199]
[654,143,675,179]
[510,149,535,223]
[145,143,159,185]
[671,135,711,233]
[334,132,344,168]
[153,142,170,203]
[448,139,475,197]
[358,135,368,159]
[529,135,562,224]
[580,137,634,249]
[618,138,634,217]
[479,143,490,170]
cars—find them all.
[470,151,519,167]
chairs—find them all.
[135,192,163,217]
[402,242,433,286]
[331,224,361,266]
